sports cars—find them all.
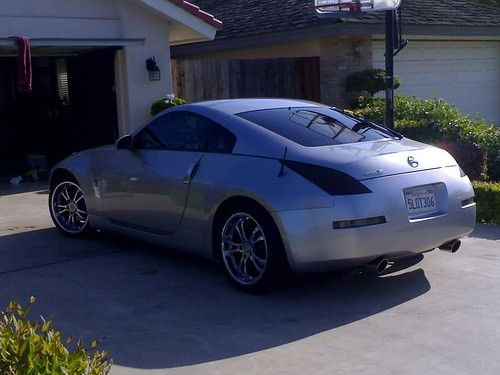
[48,99,477,298]
